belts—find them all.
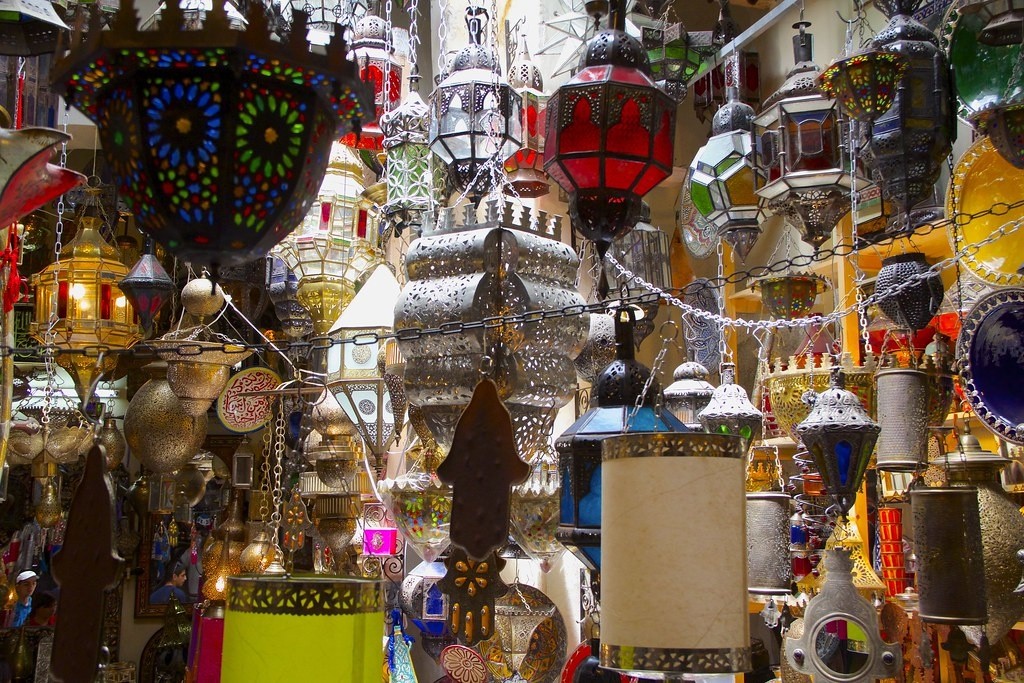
[189,592,198,597]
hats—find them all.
[17,571,39,583]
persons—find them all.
[12,568,41,628]
[148,558,190,604]
[20,591,59,628]
